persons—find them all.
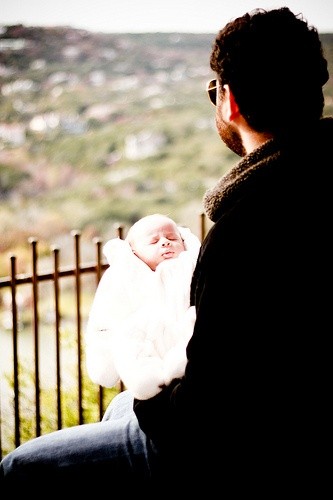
[0,6,333,500]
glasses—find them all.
[206,79,222,107]
